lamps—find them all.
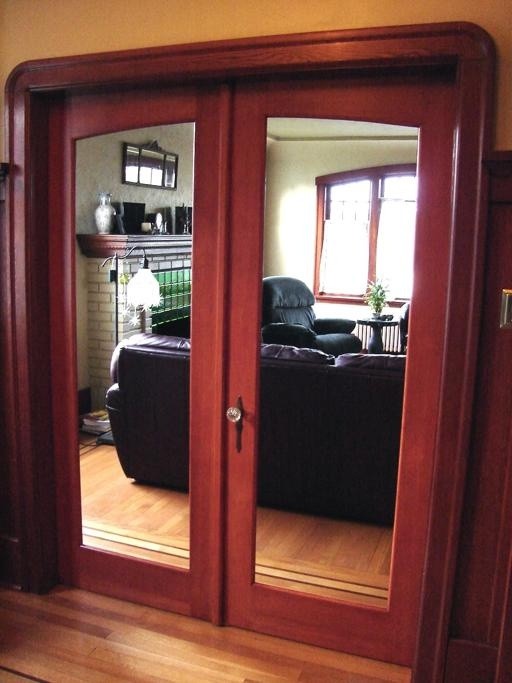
[96,245,164,445]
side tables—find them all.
[357,319,398,354]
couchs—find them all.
[105,332,406,526]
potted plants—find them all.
[363,278,390,319]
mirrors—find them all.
[122,140,178,190]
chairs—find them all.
[260,276,362,357]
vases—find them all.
[94,192,116,235]
